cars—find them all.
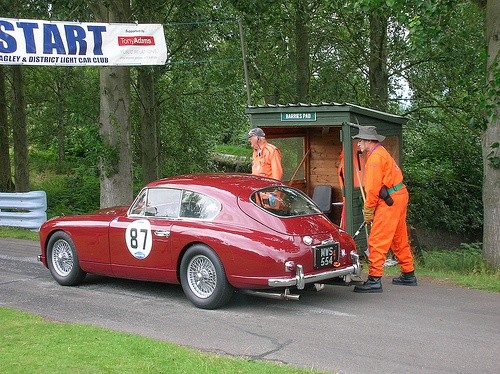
[35,172,364,311]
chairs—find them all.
[312,185,332,213]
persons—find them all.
[244,129,282,210]
[352,126,417,292]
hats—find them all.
[352,125,386,142]
[247,127,265,136]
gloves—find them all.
[363,207,375,225]
[269,193,277,206]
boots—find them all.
[392,270,417,286]
[353,275,383,293]
